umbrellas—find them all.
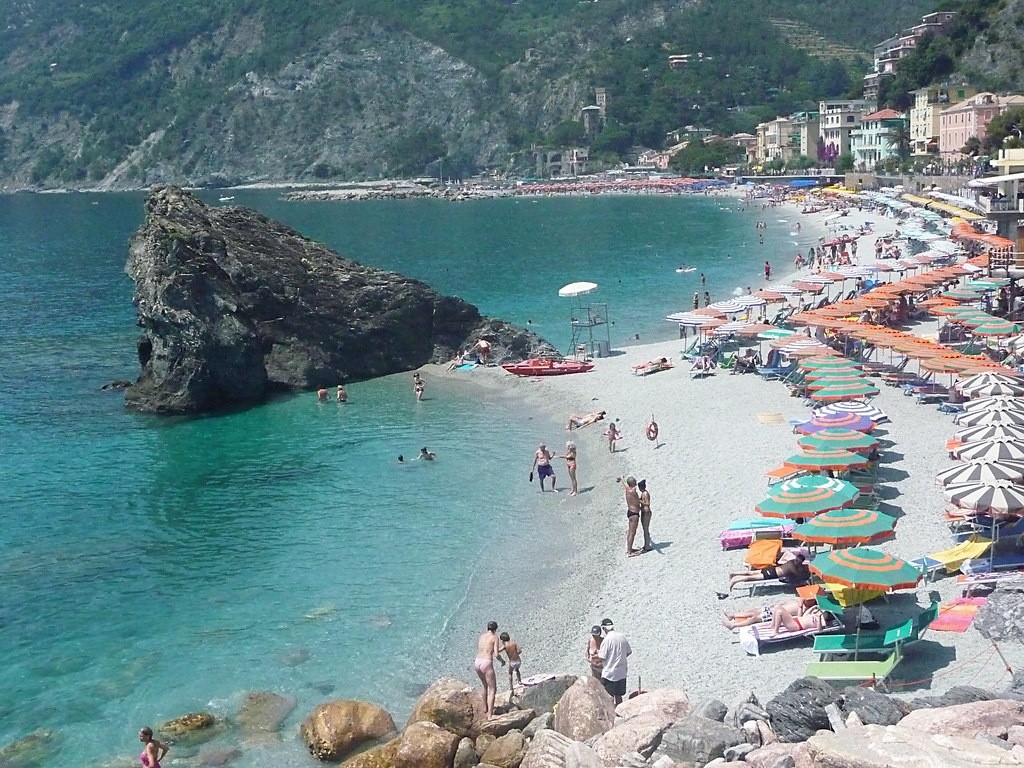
[668,188,1024,664]
[559,281,598,308]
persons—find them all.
[561,441,577,496]
[139,726,168,768]
[769,603,835,638]
[604,424,620,453]
[469,338,491,360]
[418,446,435,458]
[728,554,806,588]
[719,598,816,627]
[474,621,522,717]
[585,617,634,706]
[413,372,426,400]
[532,443,558,493]
[318,385,328,400]
[337,385,347,402]
[567,411,604,430]
[446,350,464,372]
[622,478,652,555]
[635,188,910,374]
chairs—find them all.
[636,197,1024,694]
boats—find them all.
[501,355,596,376]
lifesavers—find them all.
[646,421,658,441]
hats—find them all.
[638,480,646,488]
[591,626,602,636]
[600,619,613,626]
